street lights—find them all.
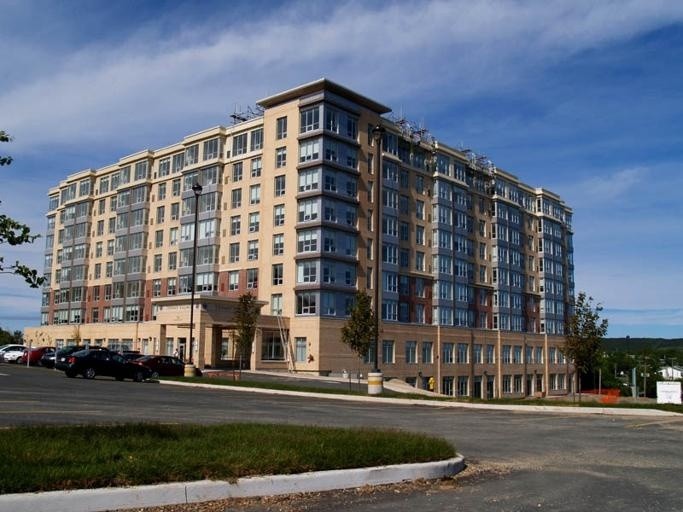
[186,181,203,365]
[372,122,384,372]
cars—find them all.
[0,344,203,382]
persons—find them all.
[174,349,179,359]
